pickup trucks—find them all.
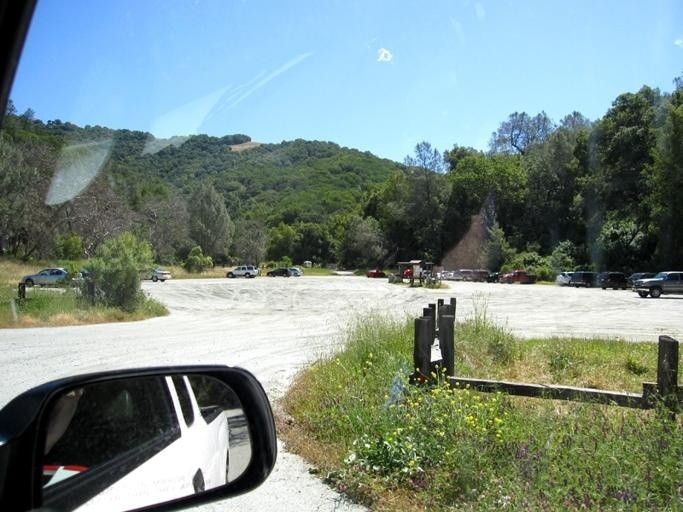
[41,375,229,512]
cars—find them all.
[422,269,536,284]
[267,267,302,277]
[556,270,683,298]
[141,268,171,282]
[367,270,383,278]
[21,268,89,287]
[226,265,258,278]
